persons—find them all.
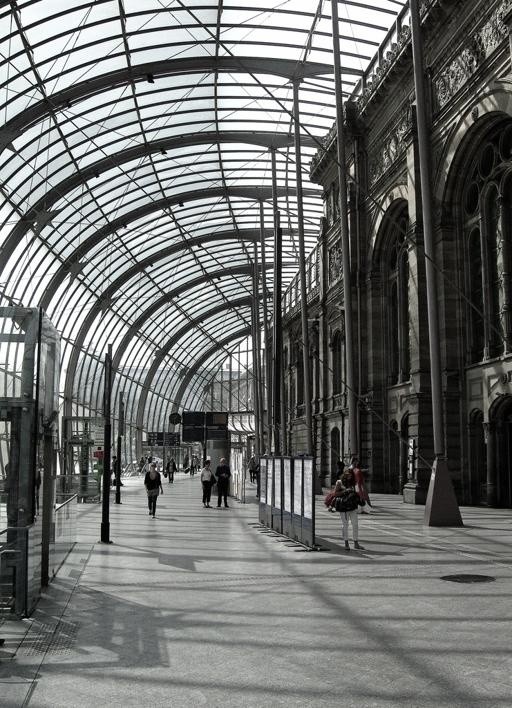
[215,457,231,507]
[324,454,371,550]
[139,455,200,483]
[199,459,214,509]
[248,453,270,499]
[112,455,121,479]
[143,463,164,518]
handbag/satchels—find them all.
[334,489,360,512]
[209,469,217,486]
[163,471,167,478]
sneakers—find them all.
[344,543,350,550]
[148,509,156,518]
[203,504,231,508]
[353,545,366,550]
